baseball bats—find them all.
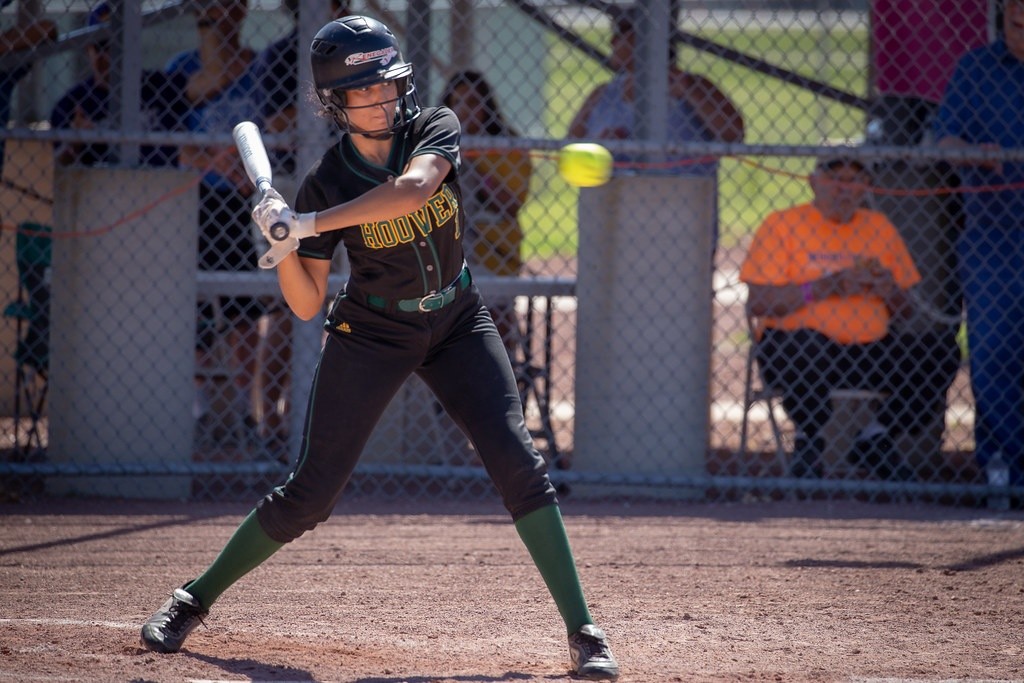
[231,120,291,242]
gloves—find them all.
[252,188,320,269]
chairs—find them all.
[741,299,883,467]
[4,220,52,455]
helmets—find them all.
[310,16,412,111]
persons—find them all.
[740,142,962,502]
[142,15,622,680]
[568,8,743,255]
[1,0,532,461]
[919,0,1024,514]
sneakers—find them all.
[568,623,618,682]
[140,579,209,654]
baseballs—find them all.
[562,140,614,188]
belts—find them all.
[368,268,470,313]
